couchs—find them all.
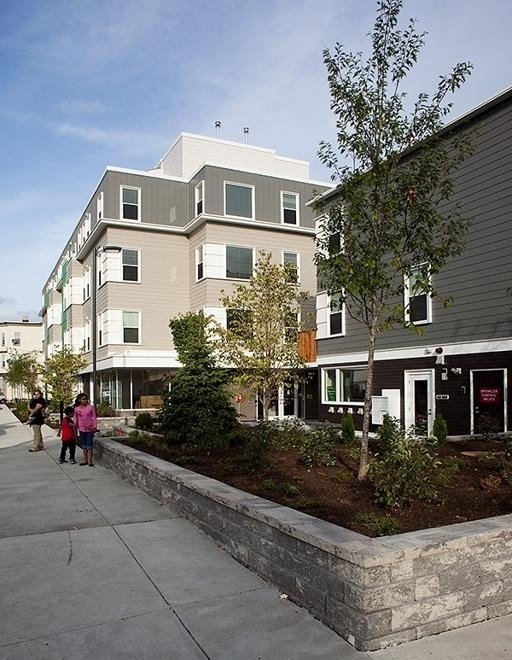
[140,395,162,408]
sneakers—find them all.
[56,457,65,464]
[69,458,76,464]
[28,446,43,452]
[79,461,94,467]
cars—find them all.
[0,392,8,405]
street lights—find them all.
[90,245,124,421]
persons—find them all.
[57,406,78,464]
[73,393,98,467]
[28,389,47,453]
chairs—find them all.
[135,401,141,409]
